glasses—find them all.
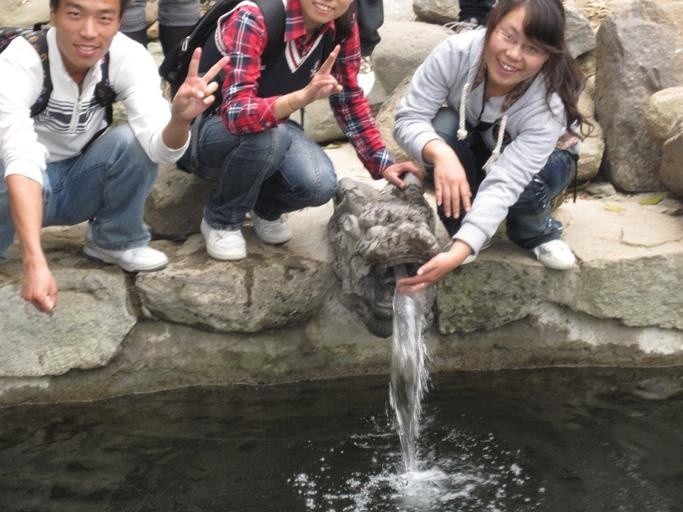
[494,28,546,58]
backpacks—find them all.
[0,22,116,153]
[159,1,284,118]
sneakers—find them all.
[530,237,576,270]
[356,55,376,98]
[81,218,168,273]
[250,208,292,247]
[200,217,246,261]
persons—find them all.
[397,0,598,293]
[171,0,425,261]
[353,0,386,96]
[0,0,232,313]
[119,0,200,60]
[441,0,497,36]
